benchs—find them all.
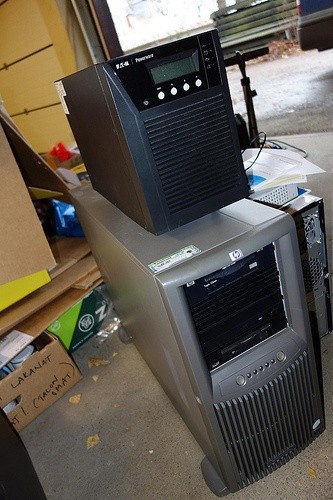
[210,0,298,61]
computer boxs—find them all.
[66,181,328,497]
[248,185,333,340]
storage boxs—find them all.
[0,330,84,434]
[46,290,119,356]
